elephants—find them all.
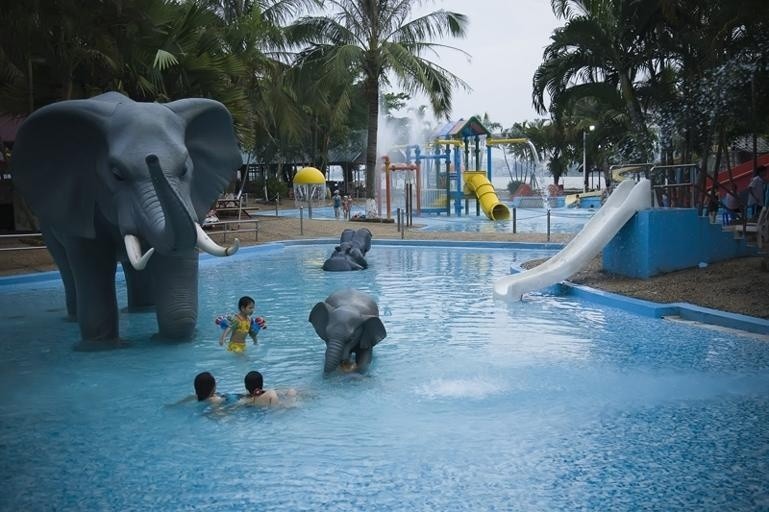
[309,288,387,380]
[9,91,243,343]
[321,228,372,271]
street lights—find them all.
[582,125,595,192]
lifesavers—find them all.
[215,313,238,330]
[249,317,266,336]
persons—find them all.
[231,369,299,409]
[747,164,767,218]
[703,182,721,222]
[162,371,255,419]
[574,195,580,205]
[351,212,360,220]
[214,296,267,354]
[725,183,743,212]
[341,195,349,218]
[599,179,613,207]
[347,193,352,211]
[331,189,342,217]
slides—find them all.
[565,165,640,205]
[494,179,650,303]
[463,170,510,221]
